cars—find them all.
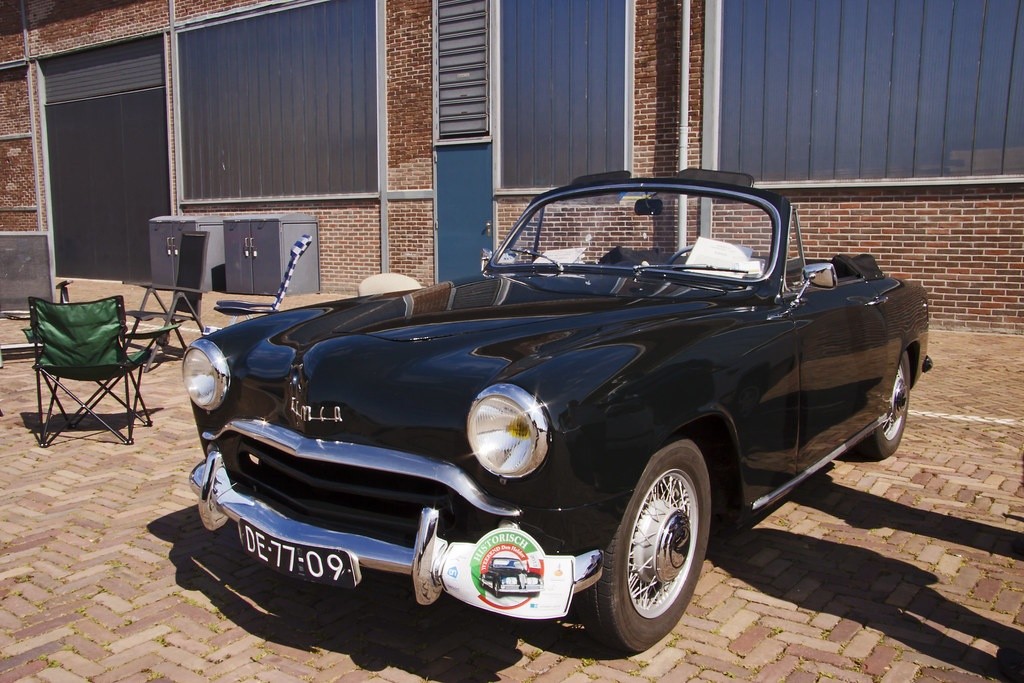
[181,167,932,656]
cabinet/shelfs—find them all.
[223,213,320,296]
[149,215,228,292]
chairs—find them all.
[28,294,152,449]
[214,234,312,325]
[125,231,210,372]
